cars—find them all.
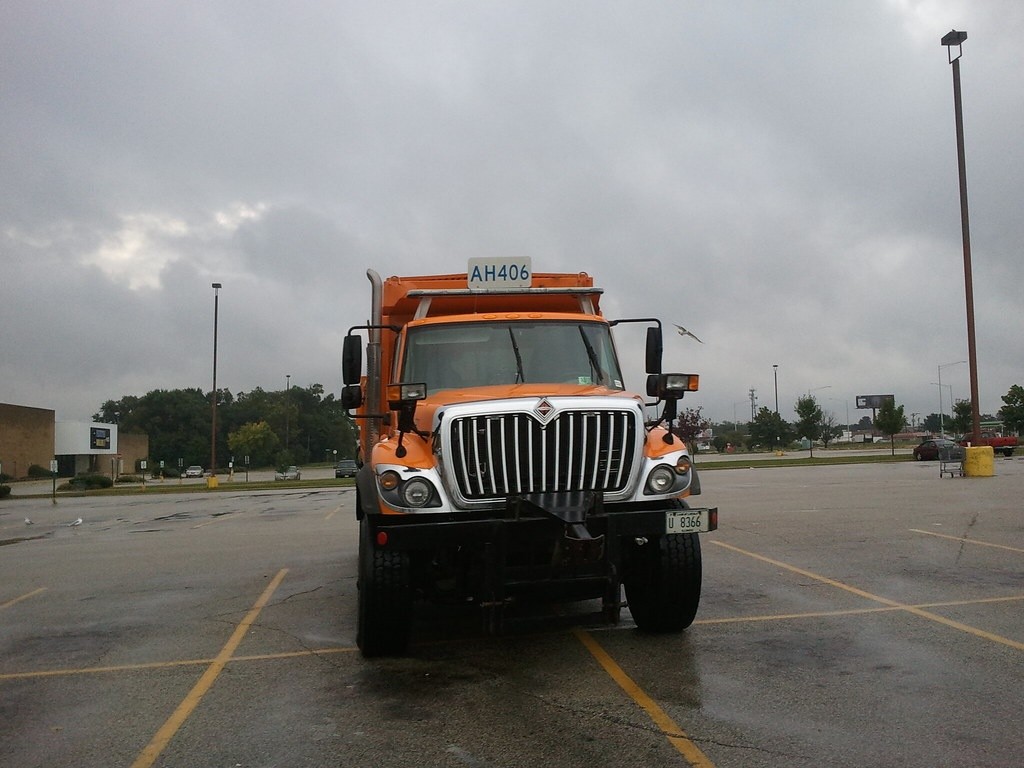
[913,439,964,461]
[274,466,301,481]
[333,460,360,478]
[185,466,204,478]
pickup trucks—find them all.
[955,430,1018,456]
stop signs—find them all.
[116,453,123,460]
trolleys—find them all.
[938,443,966,479]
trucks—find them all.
[339,257,720,656]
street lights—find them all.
[285,374,291,464]
[773,364,779,413]
[940,29,995,478]
[206,282,223,489]
